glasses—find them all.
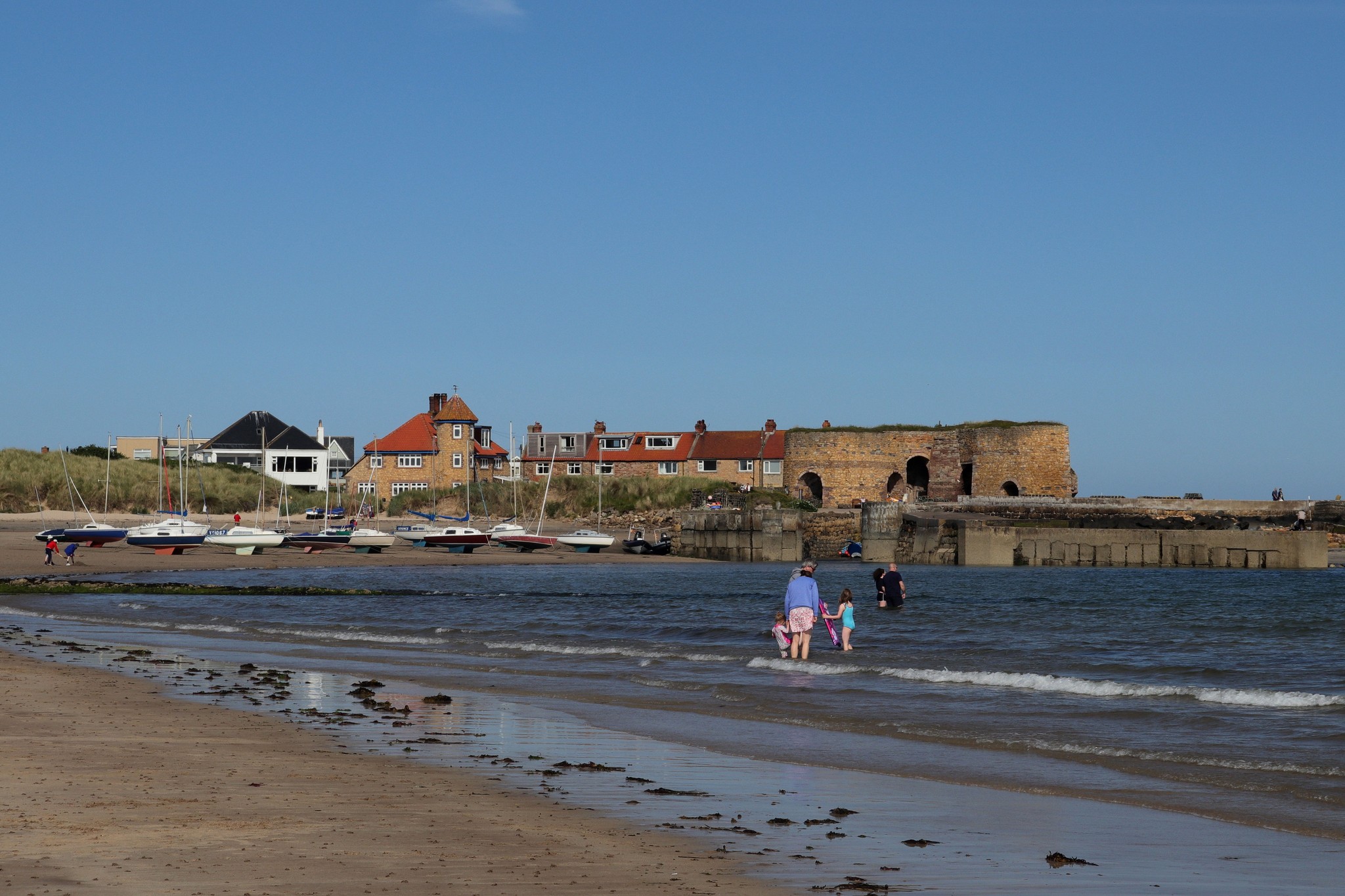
[893,567,898,569]
[883,571,887,574]
[809,566,816,572]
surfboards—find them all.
[818,598,841,648]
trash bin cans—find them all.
[901,493,908,501]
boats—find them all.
[327,506,346,520]
[305,505,325,520]
[622,522,652,555]
[652,527,672,556]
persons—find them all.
[873,563,906,608]
[822,588,855,651]
[349,517,356,532]
[64,543,79,566]
[1289,510,1306,531]
[360,503,374,519]
[738,483,751,494]
[1272,488,1285,501]
[861,496,866,508]
[234,512,241,526]
[784,558,819,658]
[44,535,60,566]
[772,611,802,658]
[372,526,376,530]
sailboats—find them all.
[122,411,538,556]
[555,448,618,554]
[34,430,129,548]
[497,444,558,555]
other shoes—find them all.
[48,563,52,566]
[51,563,55,565]
[66,563,71,566]
[44,563,47,565]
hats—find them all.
[236,511,240,514]
[75,543,80,547]
[53,540,58,543]
[47,535,53,539]
